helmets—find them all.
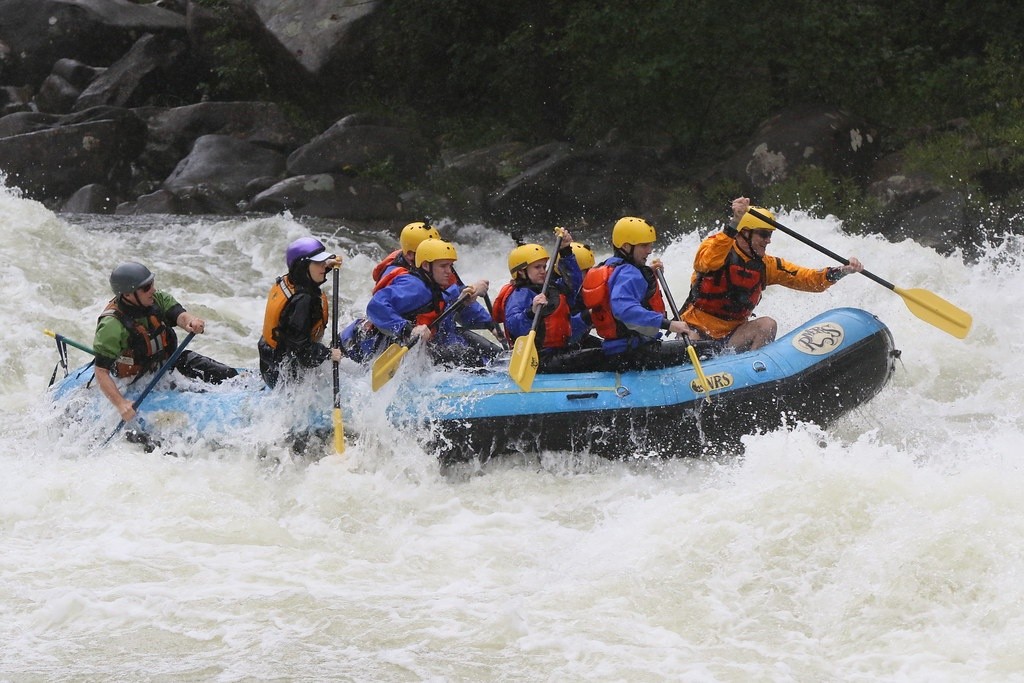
[286,237,326,268]
[554,242,595,276]
[400,223,442,255]
[736,206,776,233]
[507,244,550,280]
[415,239,457,268]
[109,262,155,300]
[611,216,657,248]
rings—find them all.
[200,325,203,327]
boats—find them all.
[47,307,902,465]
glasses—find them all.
[742,227,773,239]
[138,279,155,292]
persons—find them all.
[92,261,237,422]
[493,225,604,373]
[678,196,863,354]
[363,222,504,373]
[583,216,723,368]
[257,237,343,387]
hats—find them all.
[309,250,337,262]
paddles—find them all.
[366,290,472,393]
[736,201,975,337]
[98,324,203,443]
[651,263,712,392]
[504,227,565,393]
[480,282,508,346]
[44,328,98,356]
[329,256,346,456]
